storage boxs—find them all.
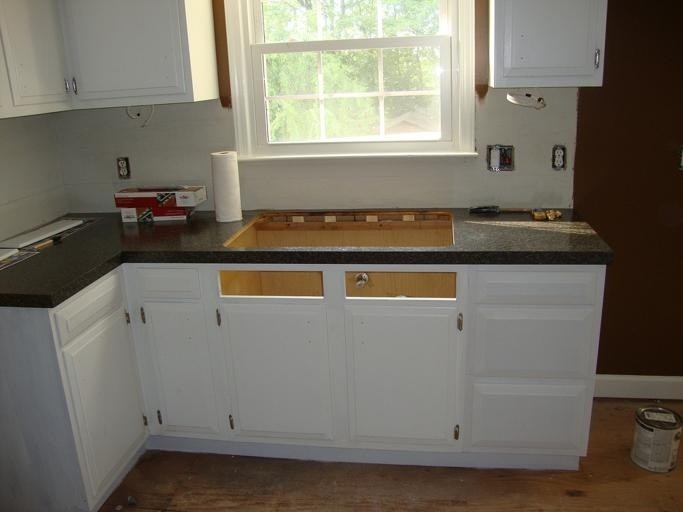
[113,185,209,223]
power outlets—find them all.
[116,157,131,180]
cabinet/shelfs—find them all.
[458,267,607,459]
[123,264,227,452]
[337,265,458,445]
[1,266,149,512]
[488,1,607,90]
[0,1,219,118]
[202,265,348,453]
